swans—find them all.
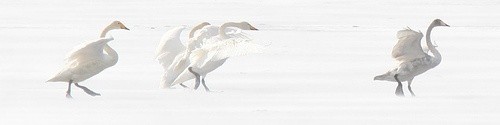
[373,19,450,96]
[171,21,259,92]
[44,20,130,100]
[157,21,251,88]
